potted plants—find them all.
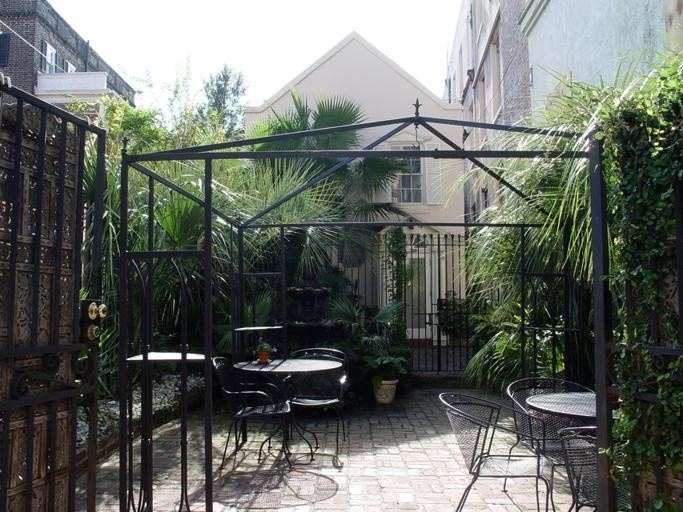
[328,294,410,404]
[257,344,271,363]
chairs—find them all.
[213,348,349,470]
[439,377,634,512]
